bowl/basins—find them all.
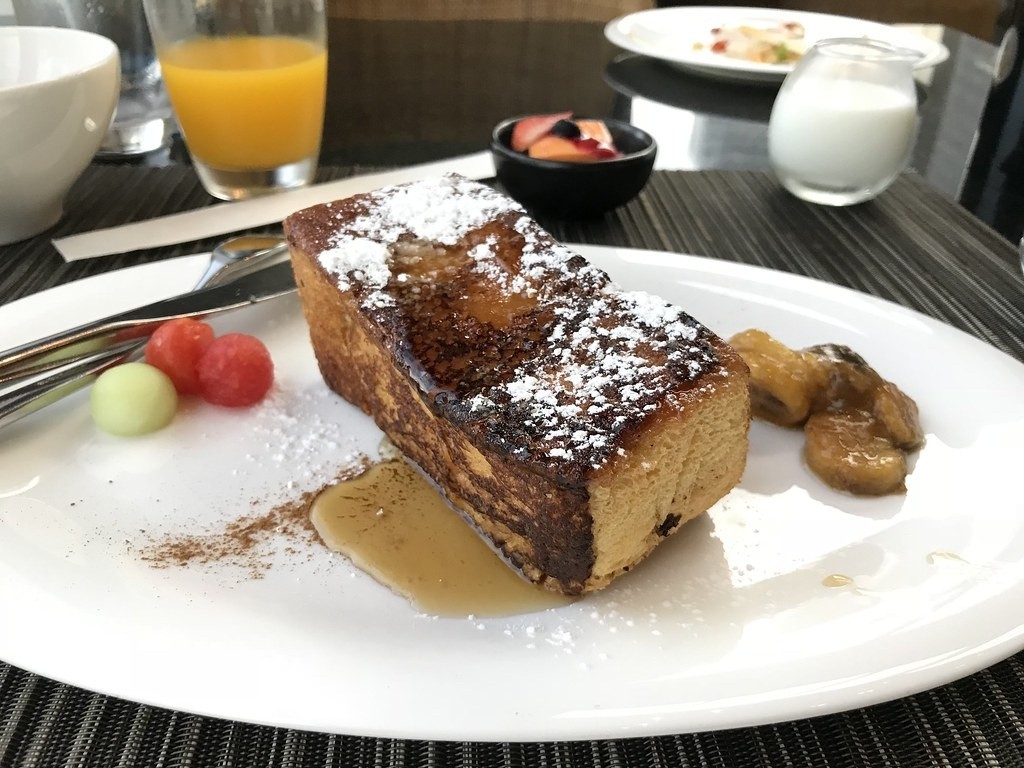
[0,28,121,244]
[489,114,657,215]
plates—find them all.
[604,6,950,81]
[1,242,1024,742]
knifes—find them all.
[0,233,292,356]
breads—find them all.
[282,171,751,594]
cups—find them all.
[0,0,174,155]
[767,38,927,204]
[141,0,329,199]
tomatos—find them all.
[144,317,274,406]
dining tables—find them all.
[2,22,1024,768]
